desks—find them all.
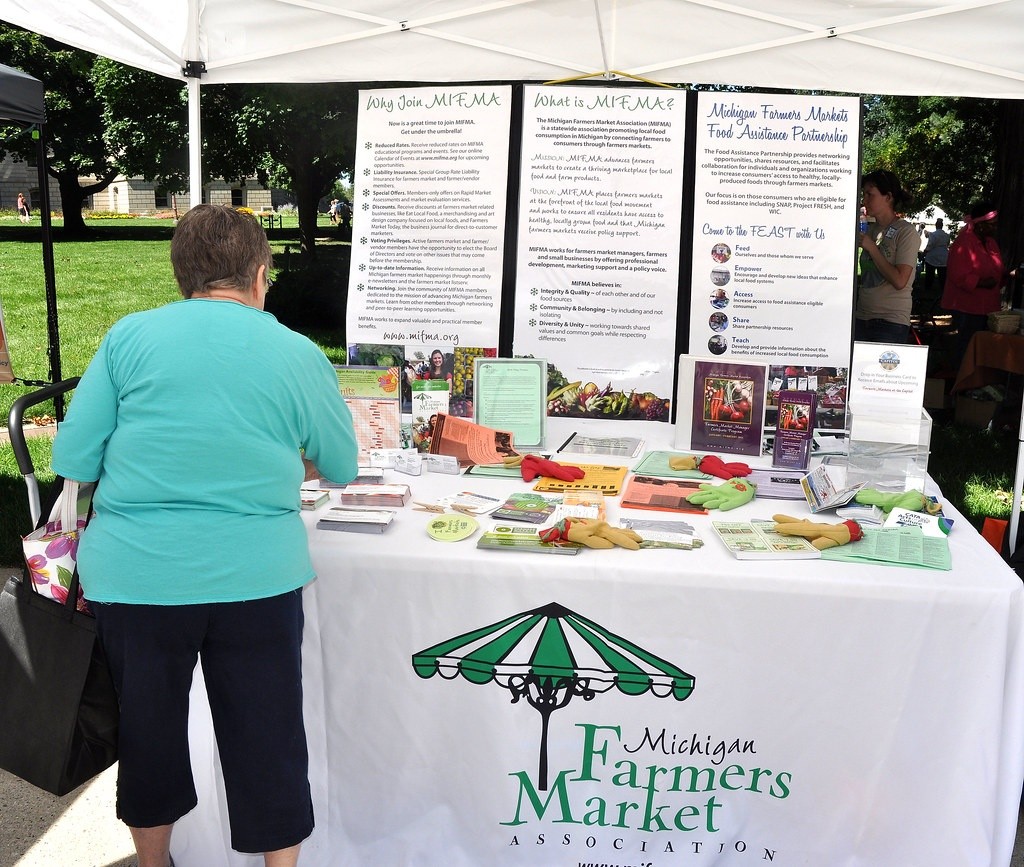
[970,330,1024,440]
[202,413,1024,867]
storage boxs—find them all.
[924,378,949,408]
[844,402,932,495]
[954,384,1019,432]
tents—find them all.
[904,205,966,252]
[0,61,63,422]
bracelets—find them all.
[980,279,984,287]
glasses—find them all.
[872,169,891,185]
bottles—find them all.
[859,209,867,234]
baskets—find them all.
[987,311,1022,334]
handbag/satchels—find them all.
[0,474,119,797]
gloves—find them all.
[539,516,642,550]
[521,454,585,482]
[669,455,752,480]
[772,515,863,550]
[685,477,757,511]
[854,488,942,520]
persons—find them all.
[425,349,452,397]
[941,201,1006,394]
[54,206,358,867]
[856,169,920,343]
[18,193,30,224]
[428,414,437,440]
[917,224,930,262]
[329,199,340,225]
[925,220,950,294]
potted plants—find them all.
[961,472,1024,553]
